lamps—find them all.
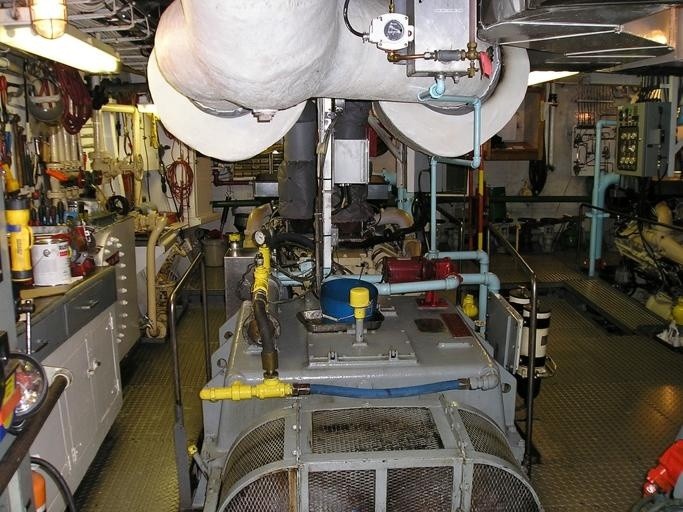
[0,0,121,75]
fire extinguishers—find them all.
[30,454,47,512]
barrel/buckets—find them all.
[321,278,378,324]
[30,233,71,286]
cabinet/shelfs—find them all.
[16,273,122,510]
